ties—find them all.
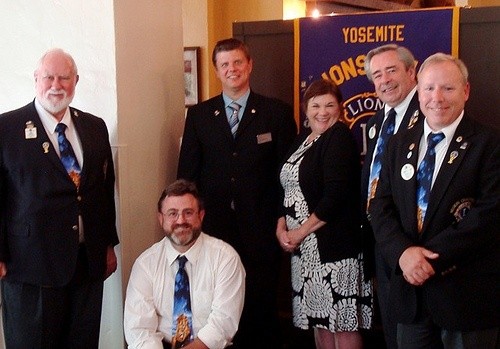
[54,123,81,193]
[172,255,192,349]
[416,133,446,235]
[366,108,397,217]
[227,102,242,139]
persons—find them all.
[368,52,500,349]
[177,38,298,349]
[0,47,120,349]
[363,43,424,349]
[123,181,246,349]
[275,80,375,349]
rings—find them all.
[284,242,289,245]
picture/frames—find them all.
[182,46,202,108]
